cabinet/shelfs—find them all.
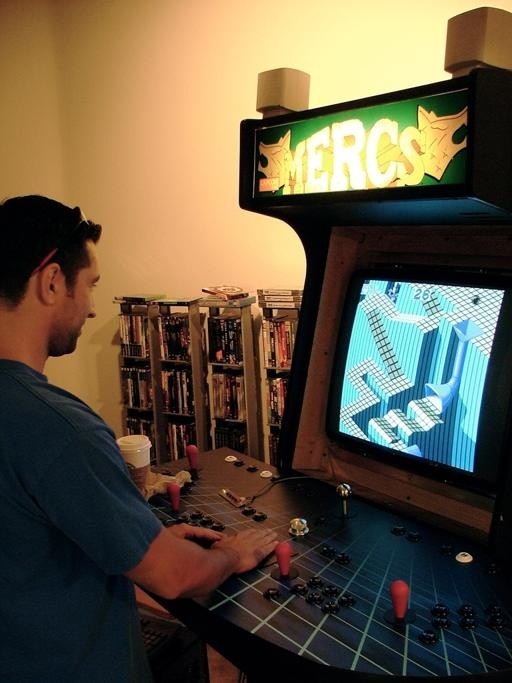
[111,296,300,466]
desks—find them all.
[136,447,510,682]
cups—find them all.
[115,434,154,489]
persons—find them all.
[0,196,278,683]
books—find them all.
[112,285,304,467]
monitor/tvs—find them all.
[324,263,512,494]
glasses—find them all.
[29,205,90,279]
